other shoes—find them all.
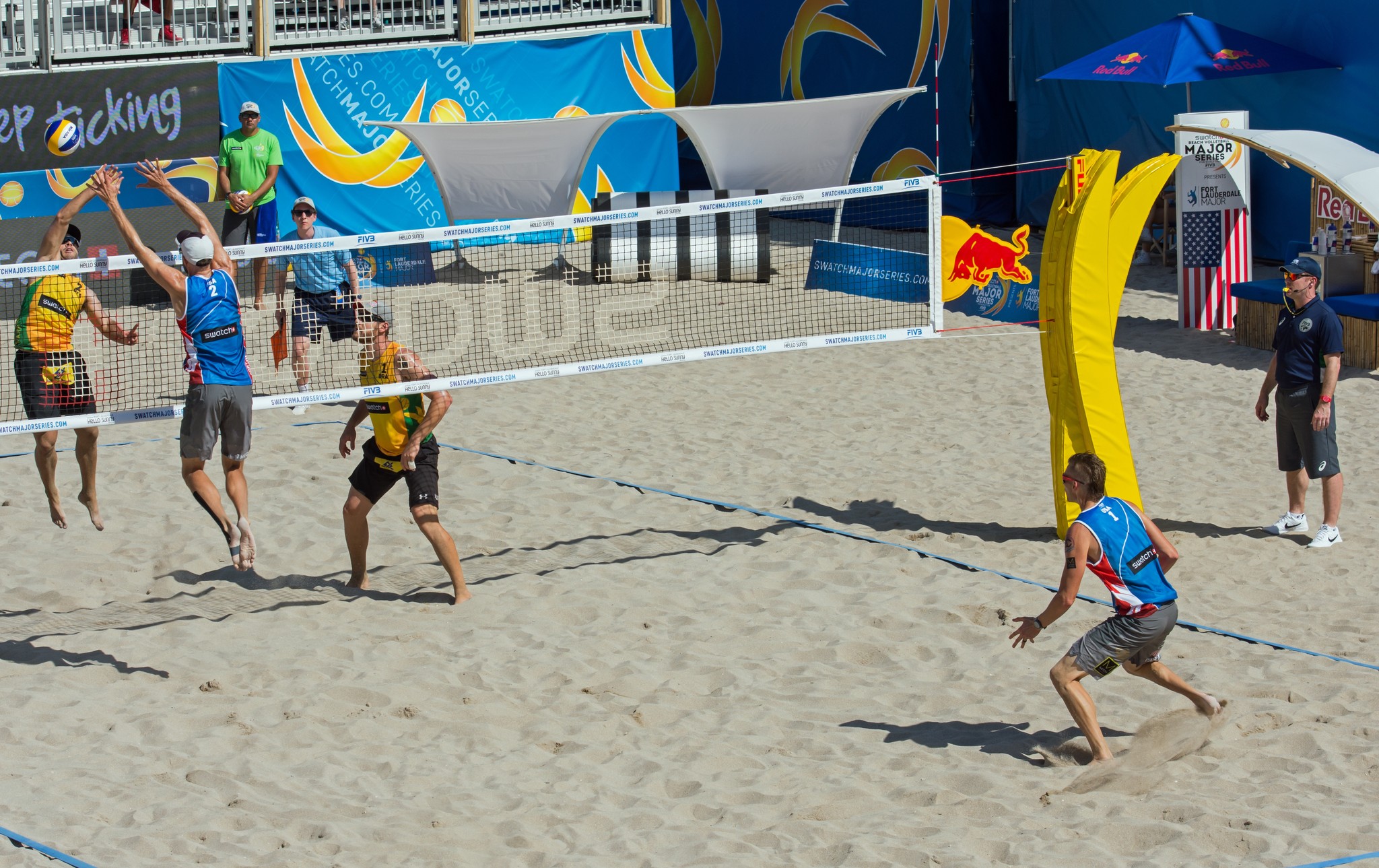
[291,404,310,416]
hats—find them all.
[352,299,393,330]
[293,197,315,210]
[176,230,214,265]
[67,224,81,242]
[239,102,260,114]
[1279,256,1322,279]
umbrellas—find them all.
[1036,11,1346,114]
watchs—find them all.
[1034,617,1047,629]
[353,295,362,299]
[1319,395,1331,402]
[224,192,232,200]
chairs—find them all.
[1133,192,1178,266]
[1230,240,1379,370]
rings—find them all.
[1022,639,1027,642]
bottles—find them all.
[1368,220,1377,233]
[1318,229,1327,255]
[1342,204,1351,226]
[1315,227,1321,237]
[1342,221,1352,253]
[1327,223,1337,255]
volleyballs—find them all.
[230,190,254,214]
[44,120,81,156]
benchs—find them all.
[0,0,655,67]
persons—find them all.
[119,0,183,46]
[559,0,584,12]
[1255,256,1344,548]
[218,101,284,310]
[274,196,362,415]
[85,158,256,573]
[14,163,140,532]
[291,0,315,3]
[1008,451,1221,766]
[329,299,473,607]
[335,0,385,30]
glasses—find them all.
[1284,272,1312,281]
[1062,473,1085,485]
[359,313,384,323]
[293,210,315,217]
[242,114,259,119]
[179,247,184,255]
[61,236,79,248]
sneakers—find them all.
[1261,511,1308,535]
[158,25,183,43]
[1306,525,1343,548]
[120,29,131,46]
